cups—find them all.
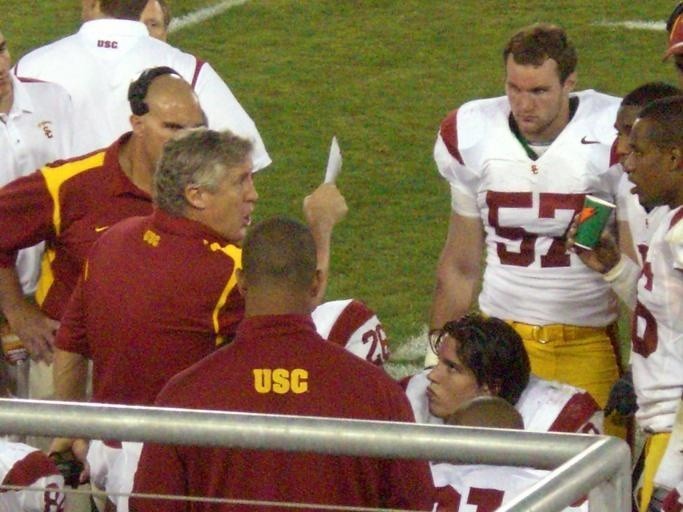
[574,195,616,250]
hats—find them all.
[661,1,683,62]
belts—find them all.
[514,321,602,339]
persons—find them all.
[569,3,682,512]
[431,22,637,437]
[55,127,349,512]
[126,216,435,512]
[316,296,605,512]
[1,0,274,512]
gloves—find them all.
[604,372,638,417]
